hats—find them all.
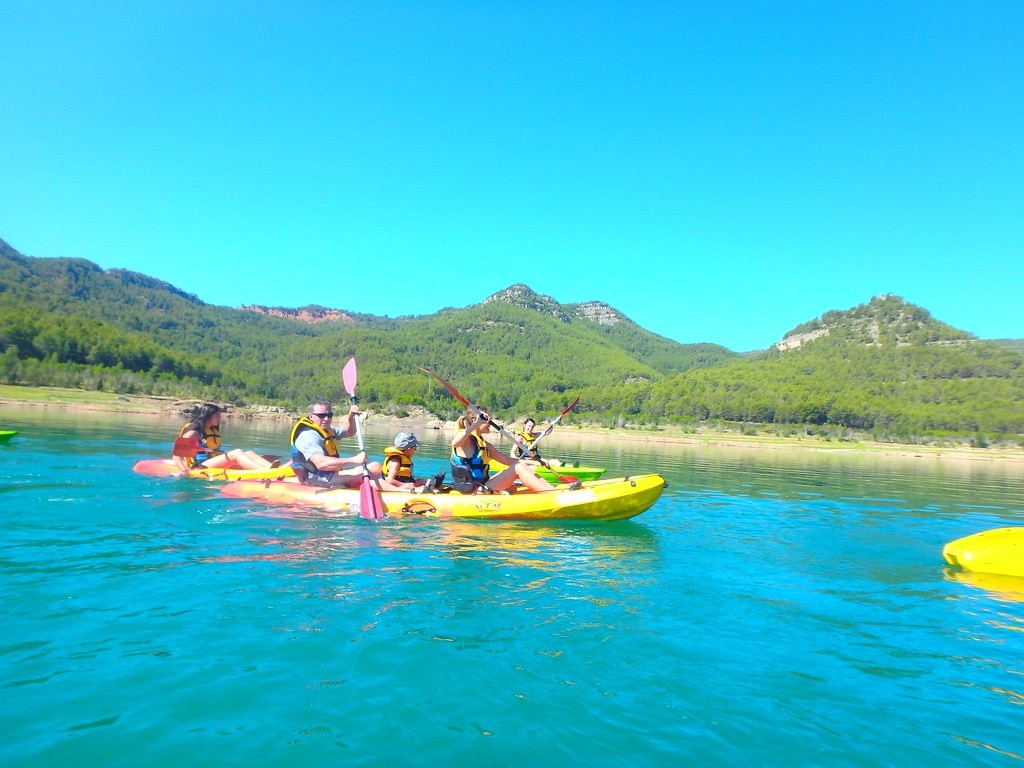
[394,431,422,450]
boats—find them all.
[0,429,18,443]
[939,562,1024,606]
[217,470,669,522]
[134,459,298,483]
[943,528,1024,576]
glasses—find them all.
[310,412,335,419]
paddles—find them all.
[517,395,581,462]
[172,437,282,464]
[340,356,385,520]
[419,367,578,484]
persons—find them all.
[511,418,580,467]
[291,400,436,493]
[451,405,581,493]
[382,432,440,493]
[173,404,292,476]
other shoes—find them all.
[270,460,281,468]
[573,460,580,469]
[560,461,567,467]
[570,480,582,491]
[420,479,436,493]
